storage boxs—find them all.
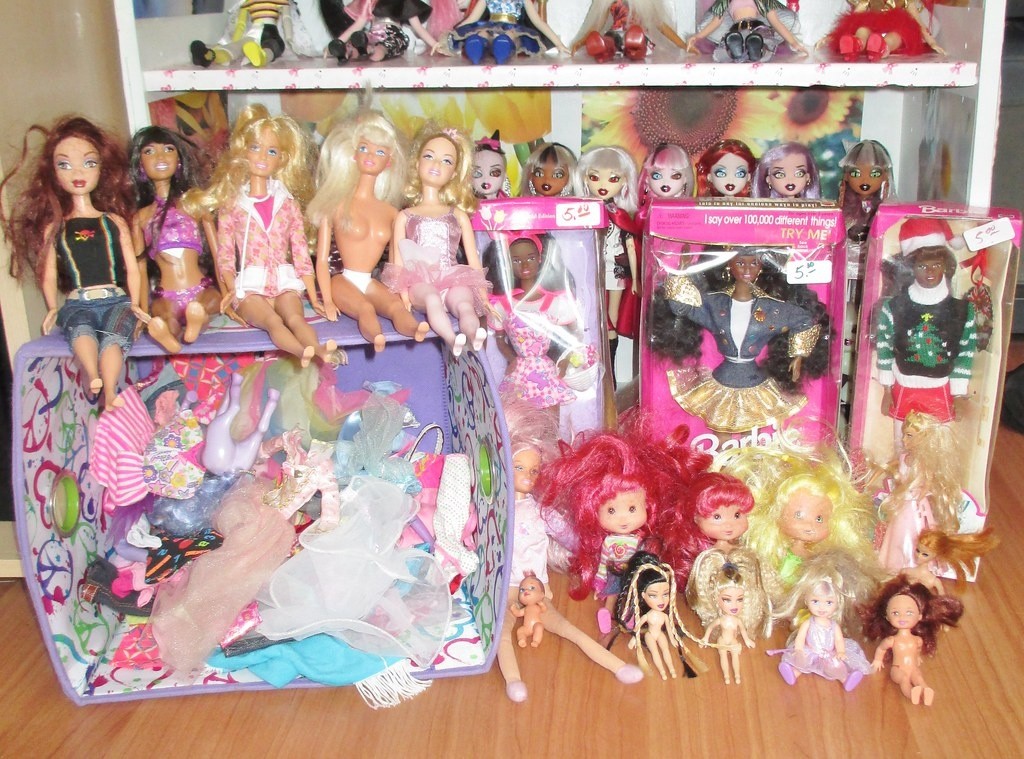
[849,204,1024,536]
[10,295,514,708]
[473,194,610,430]
[640,192,849,451]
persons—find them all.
[178,101,339,366]
[478,220,586,428]
[1,116,151,411]
[873,217,977,456]
[455,128,898,391]
[510,575,547,648]
[497,436,645,702]
[859,407,963,578]
[648,241,839,449]
[861,574,965,705]
[322,0,947,62]
[190,0,313,68]
[129,124,248,353]
[897,525,1000,633]
[301,111,431,353]
[389,123,503,356]
[531,426,872,691]
